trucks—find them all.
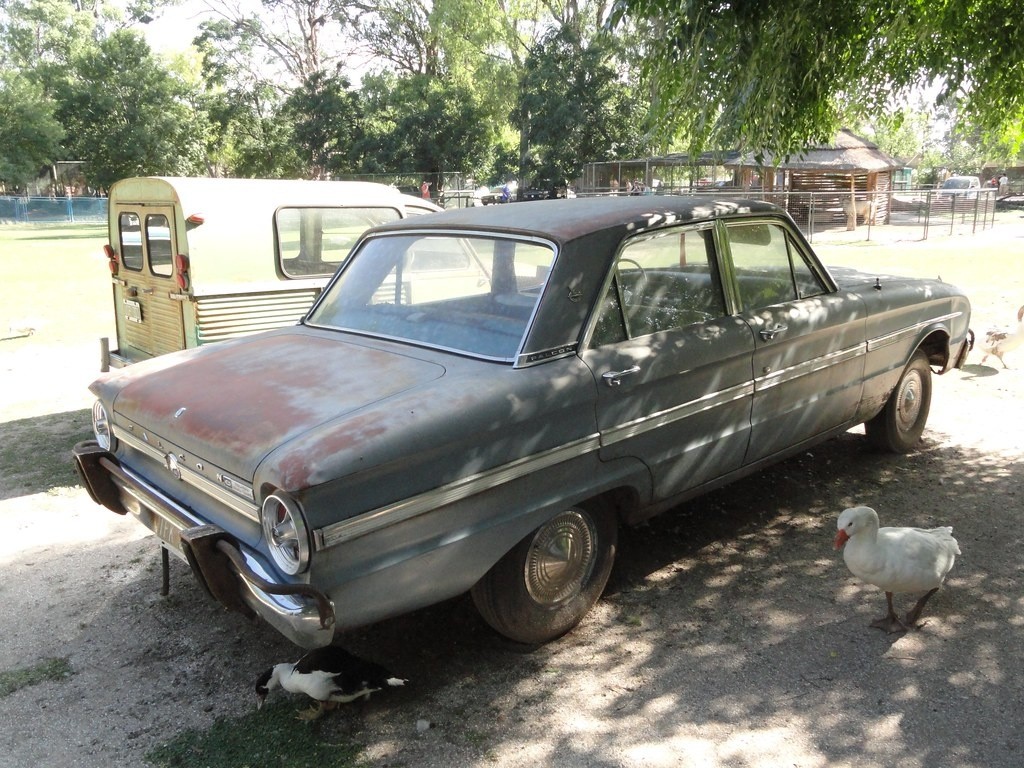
[102,176,491,375]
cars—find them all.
[72,197,977,651]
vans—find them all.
[941,177,981,201]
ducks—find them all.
[255,646,410,725]
[831,506,962,635]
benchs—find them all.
[489,268,823,349]
